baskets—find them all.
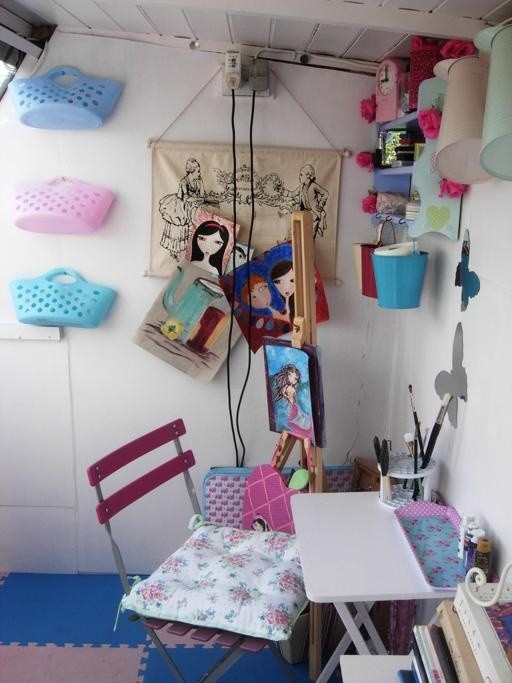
[8,265,118,329]
[11,174,117,236]
[7,64,126,130]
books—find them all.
[397,580,512,683]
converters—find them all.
[248,57,270,92]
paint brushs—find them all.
[421,392,453,483]
[403,451,410,489]
[413,422,421,501]
[408,384,424,460]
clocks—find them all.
[373,58,405,124]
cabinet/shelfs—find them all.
[371,75,463,241]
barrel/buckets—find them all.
[370,239,428,310]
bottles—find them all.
[472,537,493,583]
[466,528,485,576]
[463,522,479,568]
[456,512,476,560]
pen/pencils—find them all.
[373,435,381,463]
[380,439,391,501]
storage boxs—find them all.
[278,602,326,664]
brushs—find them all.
[403,432,414,457]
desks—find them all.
[290,492,500,683]
[339,562,511,683]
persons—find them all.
[272,363,314,439]
[190,220,295,324]
[251,514,268,531]
[159,157,215,261]
[281,164,328,238]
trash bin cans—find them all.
[278,608,309,665]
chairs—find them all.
[86,418,297,683]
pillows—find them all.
[124,524,308,641]
